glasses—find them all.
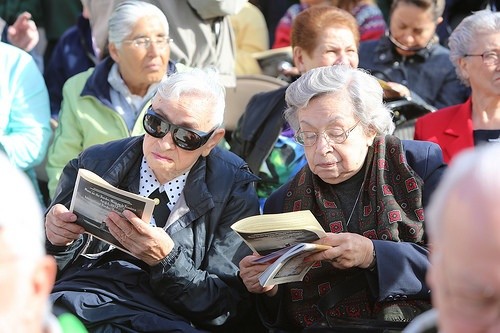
[386,18,434,52]
[295,119,360,147]
[143,104,214,150]
[121,38,173,51]
[464,51,500,65]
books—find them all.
[249,242,334,290]
[230,207,332,259]
[68,167,156,262]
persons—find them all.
[413,8,500,166]
[0,39,53,216]
[46,0,232,205]
[271,0,390,81]
[44,64,262,333]
[0,11,41,54]
[233,63,451,333]
[359,0,472,121]
[0,151,90,333]
[228,4,437,174]
[43,0,179,117]
[396,142,500,333]
[81,0,237,76]
[219,0,271,76]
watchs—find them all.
[278,59,291,73]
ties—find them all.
[148,188,171,228]
